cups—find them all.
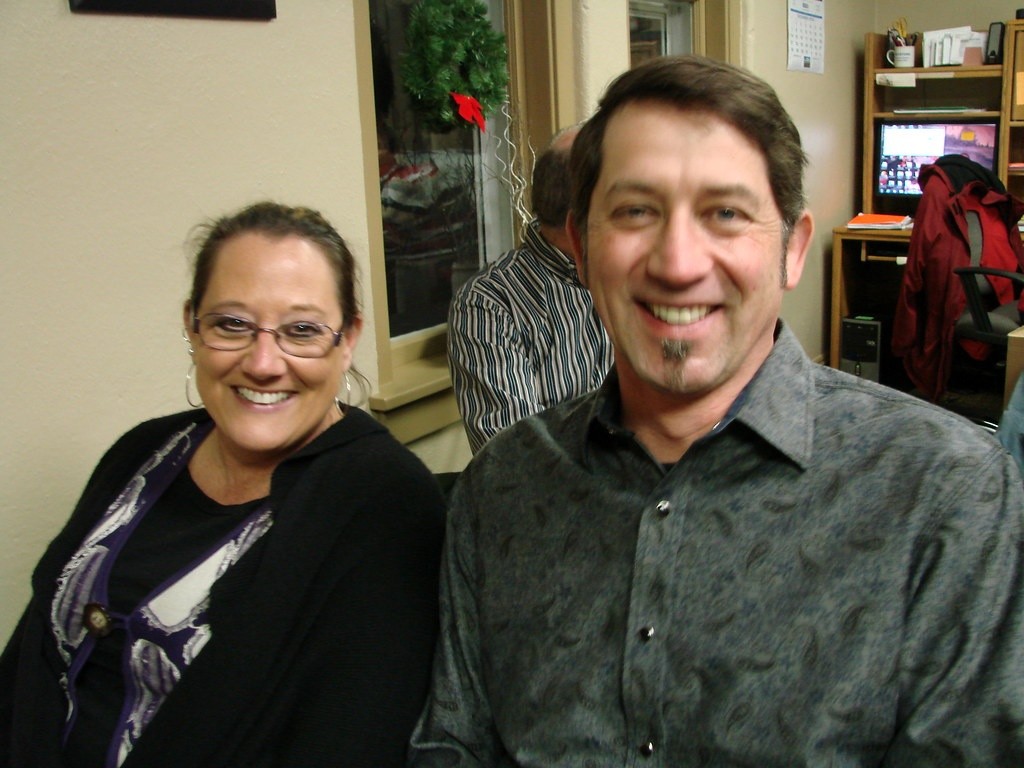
[886,46,915,67]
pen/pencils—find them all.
[887,27,918,46]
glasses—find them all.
[190,305,340,358]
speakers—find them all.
[986,22,1005,64]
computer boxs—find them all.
[839,311,892,383]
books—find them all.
[847,213,911,229]
[1010,30,1024,121]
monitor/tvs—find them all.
[874,121,1000,217]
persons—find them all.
[411,55,1024,768]
[0,202,447,768]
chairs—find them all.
[923,172,1024,432]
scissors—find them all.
[892,16,908,46]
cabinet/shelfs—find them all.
[832,20,1022,409]
